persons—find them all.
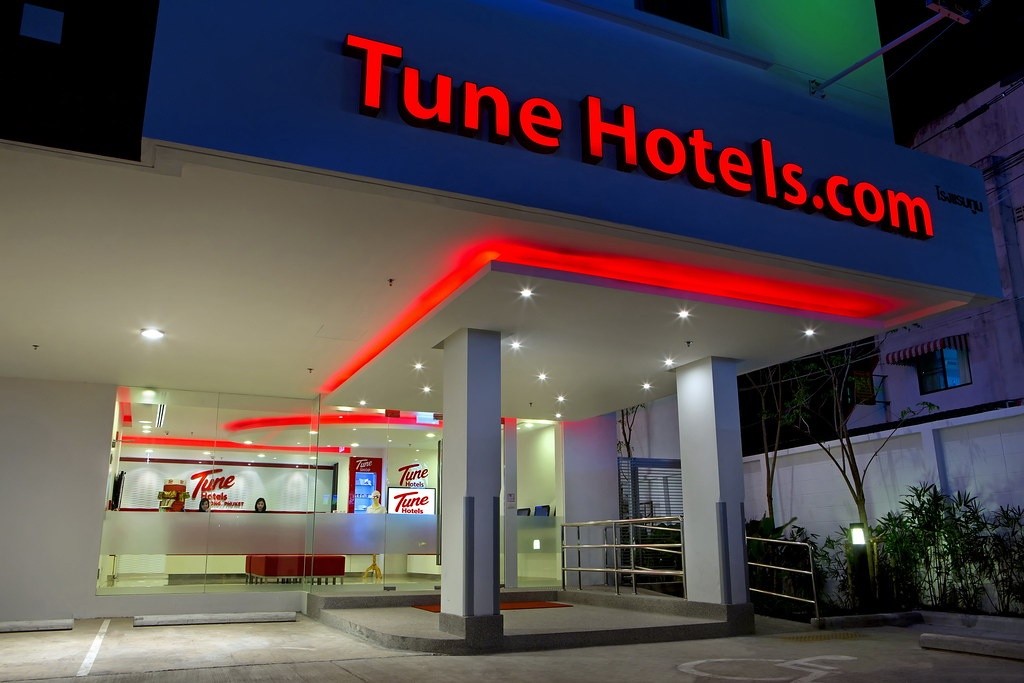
[365,490,387,514]
[198,499,212,512]
[254,498,269,513]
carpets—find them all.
[413,599,577,612]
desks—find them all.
[245,555,344,585]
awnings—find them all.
[886,334,969,367]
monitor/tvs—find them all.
[517,508,531,516]
[534,505,550,516]
[111,471,125,510]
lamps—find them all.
[850,522,866,545]
